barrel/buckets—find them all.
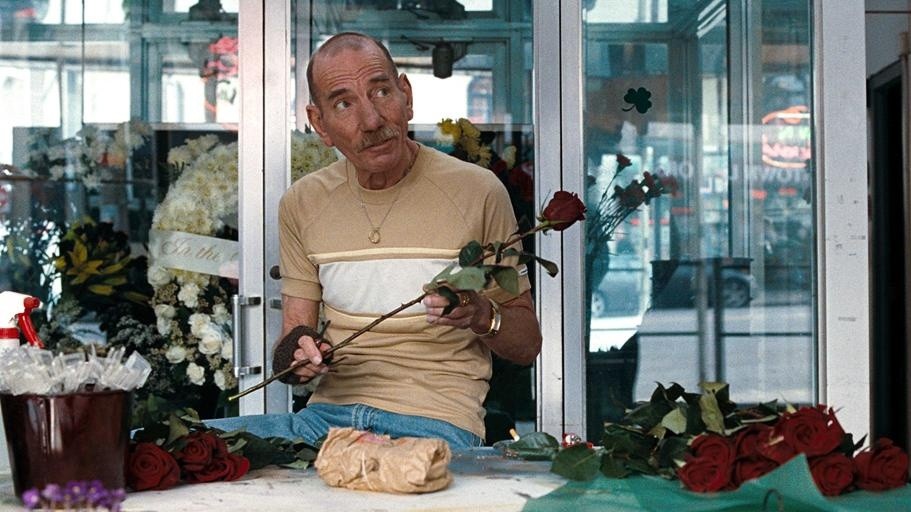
[0,387,130,501]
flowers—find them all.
[0,118,339,511]
[230,191,589,400]
[428,121,682,253]
[503,381,909,496]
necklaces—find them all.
[347,150,423,244]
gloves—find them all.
[271,325,336,386]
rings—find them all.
[459,294,470,306]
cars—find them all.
[591,255,759,318]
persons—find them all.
[179,29,543,461]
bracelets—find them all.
[471,296,505,341]
